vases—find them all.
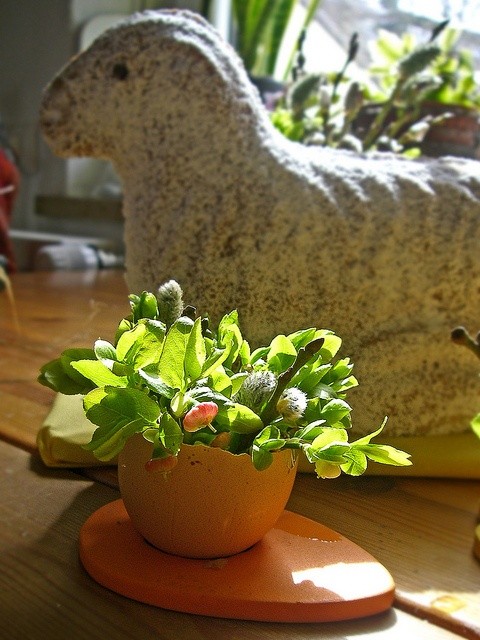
[117,431,298,559]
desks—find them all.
[0,269,480,639]
[1,436,477,640]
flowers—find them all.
[36,281,413,480]
